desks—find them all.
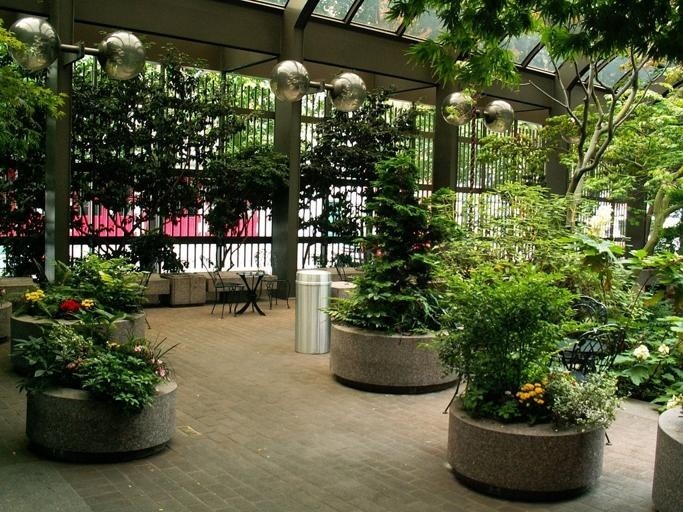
[235,270,267,315]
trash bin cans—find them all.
[294,269,331,354]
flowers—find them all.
[19,252,183,409]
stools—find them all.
[262,278,290,310]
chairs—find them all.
[200,255,242,317]
